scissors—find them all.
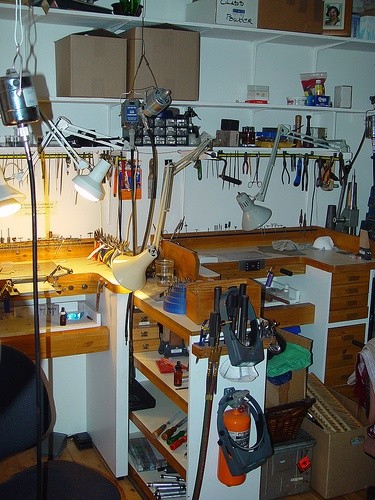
[248,151,262,188]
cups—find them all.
[241,132,256,146]
[153,259,174,286]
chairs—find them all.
[0,345,126,500]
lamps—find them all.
[111,136,210,291]
[38,108,111,202]
[236,123,350,232]
[0,116,136,220]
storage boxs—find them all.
[247,84,269,103]
[302,385,375,499]
[133,311,161,352]
[351,8,375,40]
[118,23,200,100]
[335,85,352,108]
[266,329,313,408]
[260,429,316,500]
[185,278,261,325]
[310,127,328,148]
[54,28,129,98]
[258,0,325,34]
[186,0,259,28]
[216,129,239,148]
[142,109,189,146]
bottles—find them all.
[315,80,323,96]
[174,361,183,387]
[60,307,66,326]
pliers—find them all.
[242,152,249,175]
[302,153,309,191]
[281,151,291,185]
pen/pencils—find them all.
[146,473,187,500]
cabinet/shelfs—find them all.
[0,4,375,157]
[0,225,375,500]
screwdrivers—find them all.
[151,410,187,451]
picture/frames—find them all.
[322,0,353,37]
